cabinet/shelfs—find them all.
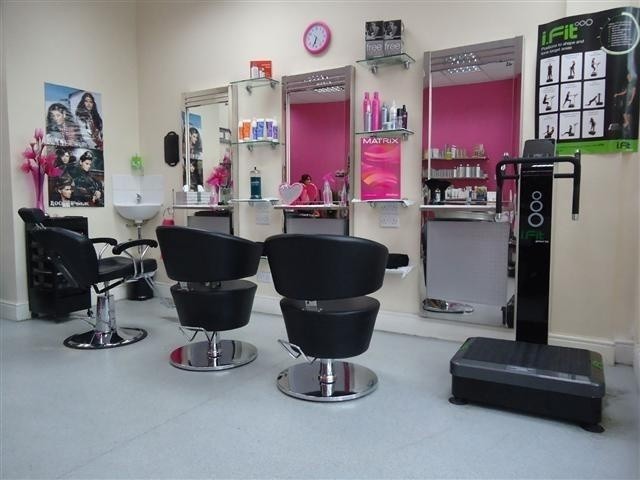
[24,215,94,323]
[356,53,415,211]
[424,155,489,182]
[228,76,281,206]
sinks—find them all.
[114,203,162,223]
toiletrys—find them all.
[431,143,485,160]
[431,164,482,178]
[249,166,261,199]
[238,118,279,143]
[425,145,488,202]
[363,91,408,131]
[445,184,478,201]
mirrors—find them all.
[281,63,355,203]
[178,83,234,205]
[420,31,523,330]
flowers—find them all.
[205,163,228,187]
[22,128,62,180]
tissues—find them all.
[175,184,198,206]
[197,185,211,205]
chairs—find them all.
[19,207,161,350]
[149,221,267,372]
[267,226,389,401]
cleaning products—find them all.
[340,175,349,205]
[322,173,335,205]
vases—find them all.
[34,172,44,213]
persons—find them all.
[386,20,401,40]
[47,92,103,206]
[189,127,202,152]
[190,159,200,190]
[299,175,317,201]
[614,73,637,131]
[369,21,383,40]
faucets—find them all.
[136,193,141,201]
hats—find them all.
[80,151,96,160]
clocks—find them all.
[304,20,331,55]
